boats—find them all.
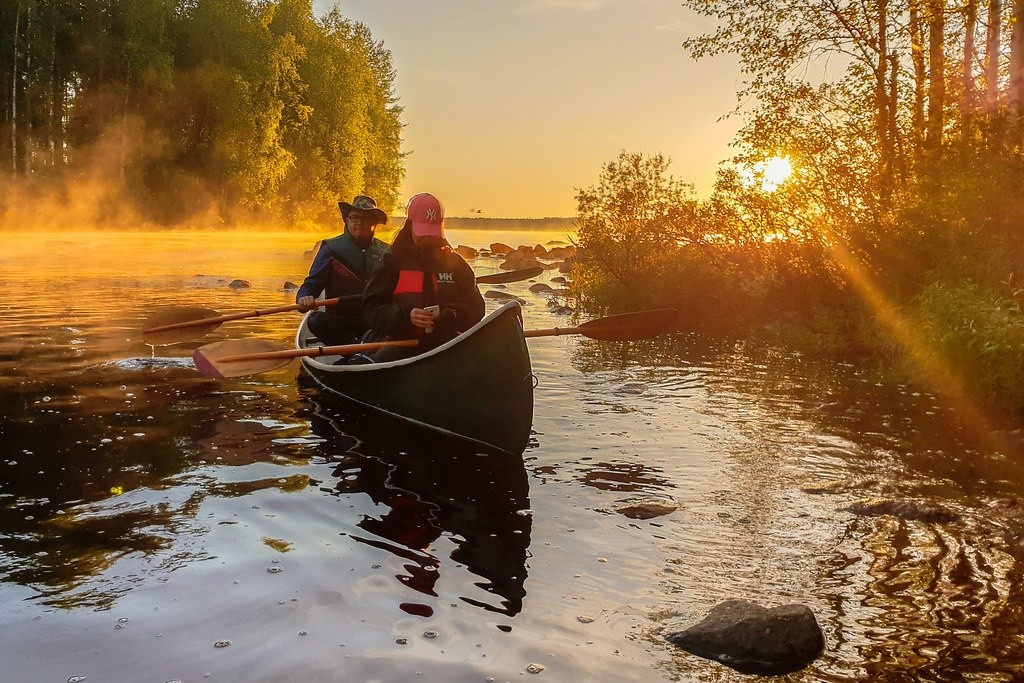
[294,288,536,466]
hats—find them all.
[338,195,387,227]
[406,193,445,237]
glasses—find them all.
[344,213,377,225]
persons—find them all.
[361,191,486,367]
[295,196,391,357]
